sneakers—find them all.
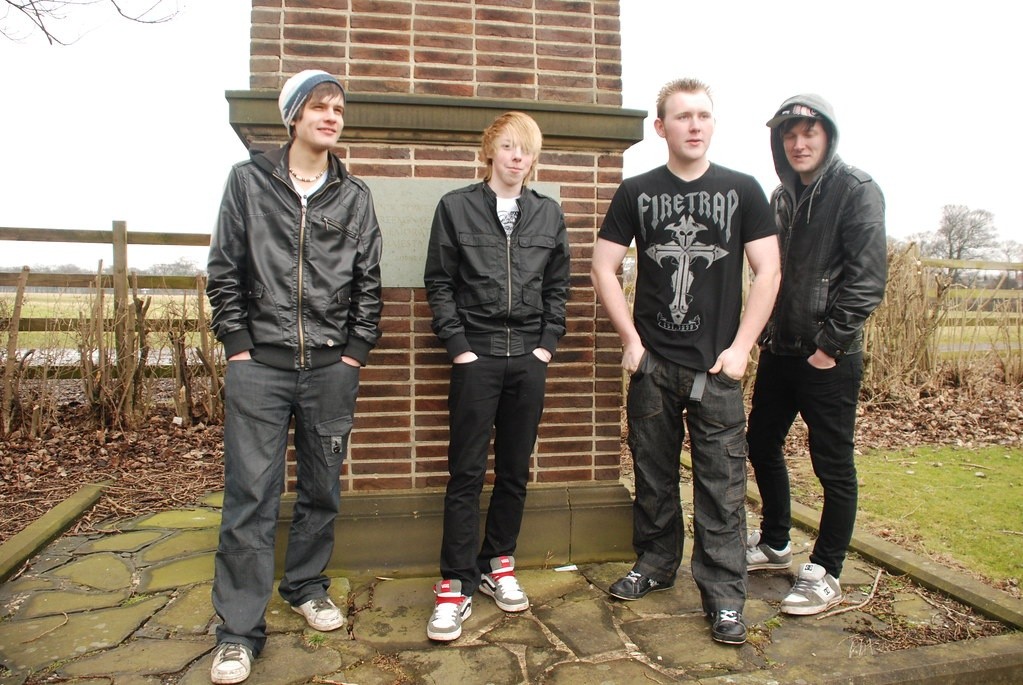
[707,611,747,646]
[209,643,252,685]
[780,562,842,616]
[426,580,471,640]
[745,531,792,571]
[476,556,528,612]
[608,568,676,601]
[290,592,344,632]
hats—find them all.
[277,69,345,139]
[765,102,825,129]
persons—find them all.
[203,68,384,682]
[590,77,785,645]
[746,92,892,619]
[422,110,573,644]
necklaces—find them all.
[285,157,330,183]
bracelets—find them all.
[541,346,552,362]
